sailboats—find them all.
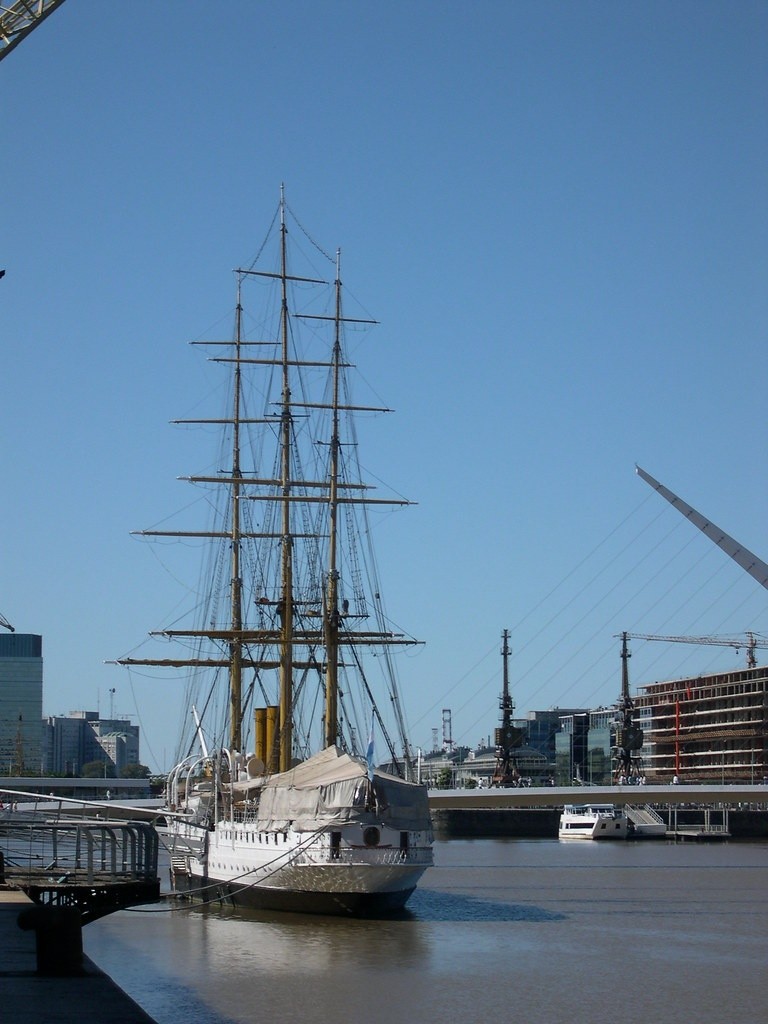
[102,173,439,921]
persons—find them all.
[50,792,54,803]
[479,773,768,810]
[96,813,100,821]
[0,790,40,812]
[106,790,110,800]
[161,787,166,798]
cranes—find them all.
[613,628,768,669]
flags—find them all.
[364,714,373,780]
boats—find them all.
[558,776,629,841]
